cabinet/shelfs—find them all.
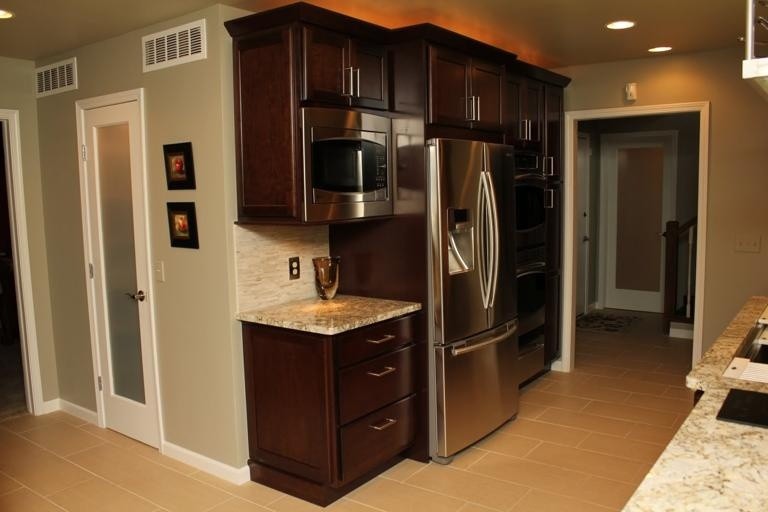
[237,292,422,508]
[392,22,517,135]
[222,1,391,226]
[518,58,572,180]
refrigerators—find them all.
[424,137,519,465]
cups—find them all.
[312,256,342,301]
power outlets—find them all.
[734,237,761,252]
[289,257,301,280]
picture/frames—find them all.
[165,202,199,250]
[162,141,197,191]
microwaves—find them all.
[299,107,393,222]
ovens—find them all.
[513,249,561,384]
[515,151,556,248]
